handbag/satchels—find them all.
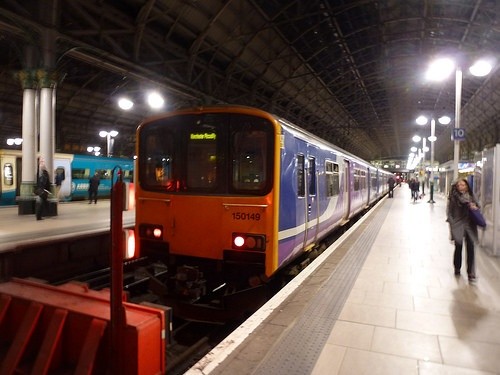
[470,209,486,226]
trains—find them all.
[0,148,135,207]
[131,107,397,306]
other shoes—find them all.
[455,267,460,274]
[468,273,476,281]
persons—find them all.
[445,179,482,281]
[399,176,420,201]
[36,170,51,221]
[88,172,101,205]
[387,176,396,198]
